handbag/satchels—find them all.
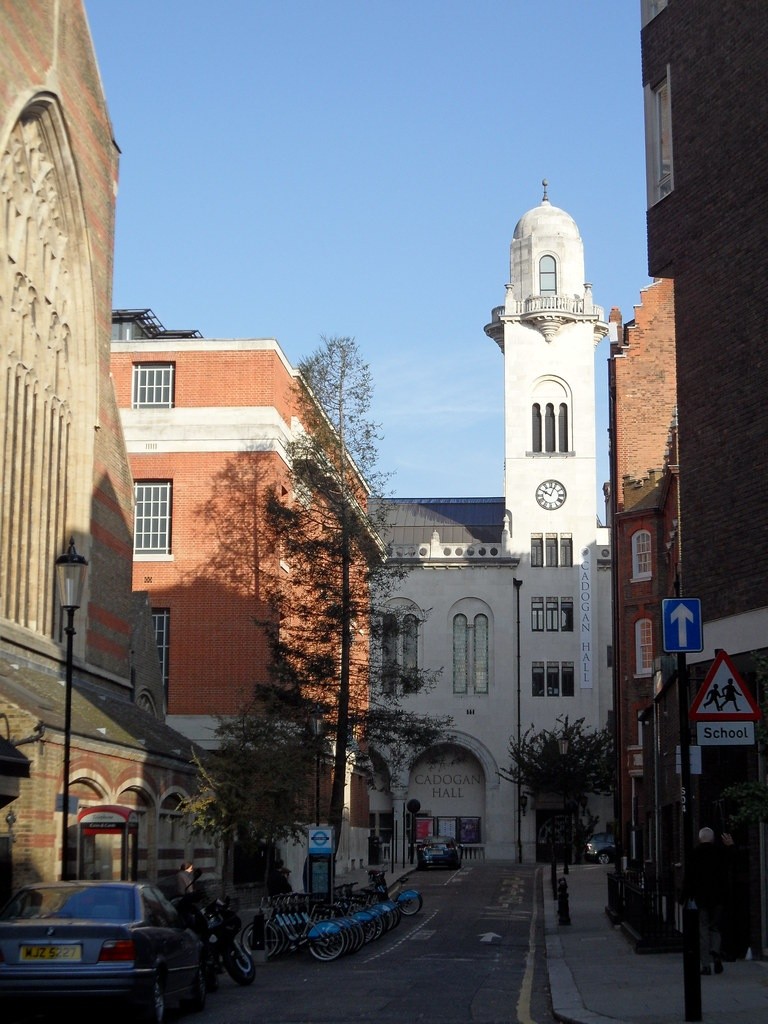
[745,947,752,960]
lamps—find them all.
[580,793,588,816]
[520,793,527,816]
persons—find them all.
[671,828,744,975]
[178,862,194,895]
[266,858,293,899]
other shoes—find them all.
[700,964,711,975]
[708,950,724,974]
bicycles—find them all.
[239,868,425,963]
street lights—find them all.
[53,536,93,881]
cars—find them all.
[583,831,614,865]
[0,877,213,1023]
[416,834,465,871]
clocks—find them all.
[535,479,567,511]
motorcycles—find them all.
[157,862,256,988]
